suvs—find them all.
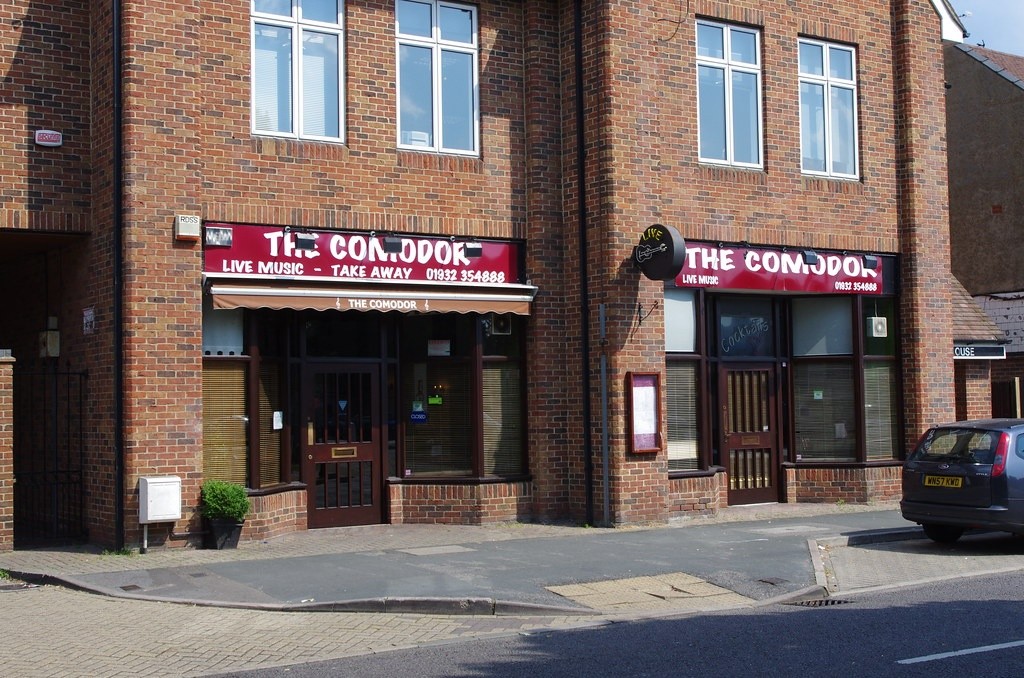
[899,417,1024,551]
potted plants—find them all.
[201,479,253,550]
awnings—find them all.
[200,271,539,316]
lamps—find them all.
[862,252,877,269]
[802,248,817,265]
[294,228,315,249]
[383,232,402,253]
[463,237,482,258]
